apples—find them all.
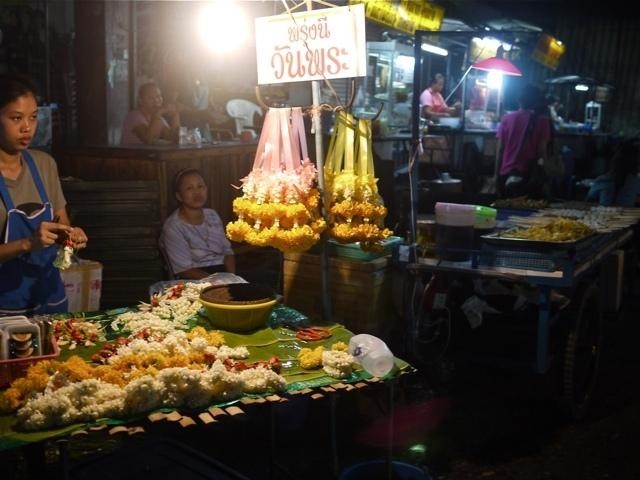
[241,131,253,142]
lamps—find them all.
[470,37,522,90]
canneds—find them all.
[175,126,187,145]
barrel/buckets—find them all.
[340,461,424,480]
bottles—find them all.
[435,201,497,261]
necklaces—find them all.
[181,205,212,248]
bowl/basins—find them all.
[196,283,278,332]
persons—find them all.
[545,94,565,124]
[584,143,640,209]
[0,76,88,319]
[420,73,470,120]
[159,167,254,302]
[119,83,181,146]
[492,83,551,202]
